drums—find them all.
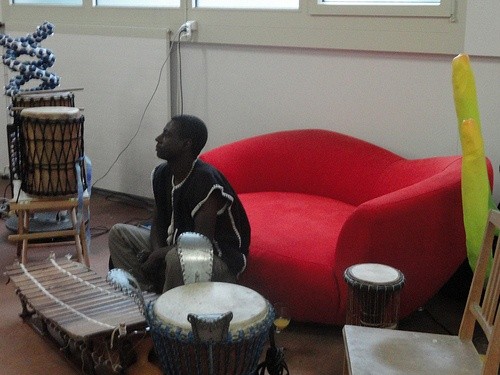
[344,261,408,329]
[142,280,276,375]
[12,91,75,108]
[20,105,85,199]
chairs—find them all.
[343,210,500,375]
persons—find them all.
[108,114,251,367]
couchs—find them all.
[196,129,493,331]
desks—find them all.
[8,177,90,270]
